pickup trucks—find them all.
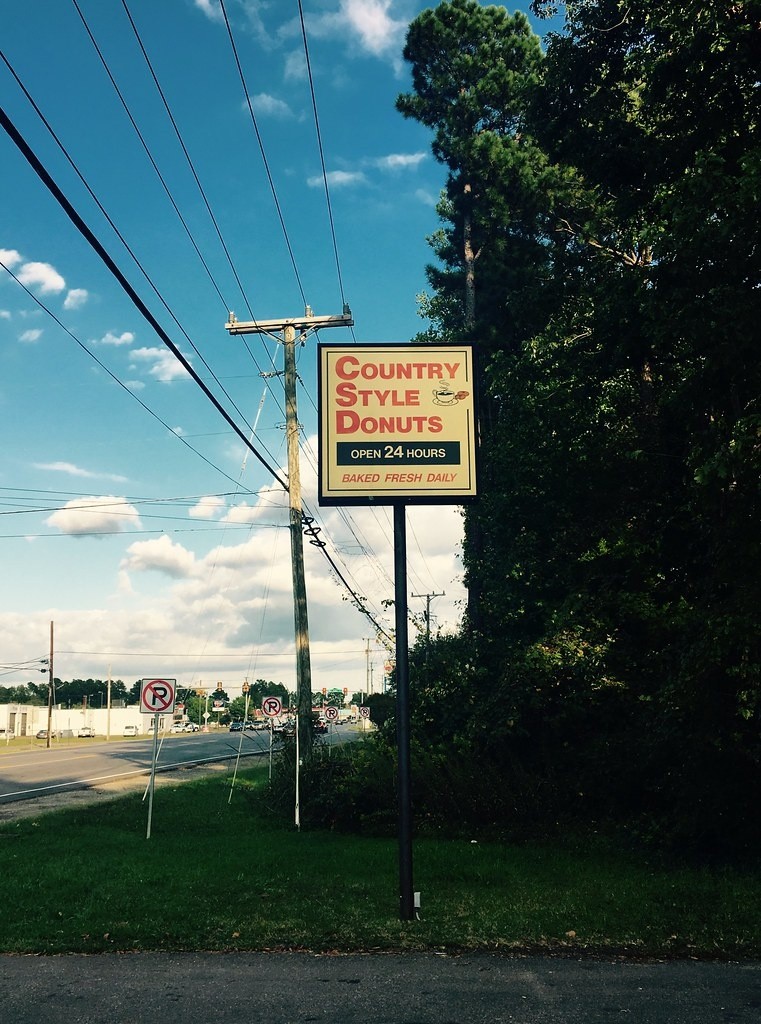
[230,723,246,732]
[78,727,95,738]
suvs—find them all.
[244,721,252,729]
[251,720,265,730]
[182,722,195,732]
[0,729,15,740]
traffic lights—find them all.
[323,688,326,696]
[344,688,347,695]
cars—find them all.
[264,715,362,735]
[147,728,160,735]
[36,730,55,739]
[172,725,192,733]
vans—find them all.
[123,725,138,737]
